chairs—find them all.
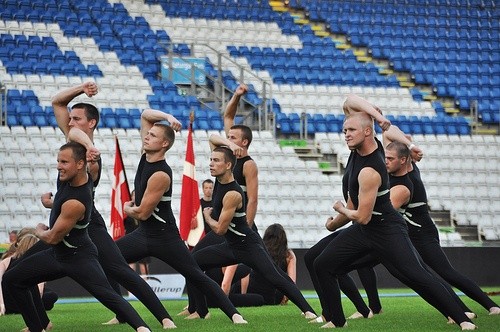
[0,0,500,244]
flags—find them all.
[178,125,208,252]
[108,140,134,242]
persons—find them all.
[0,78,500,332]
